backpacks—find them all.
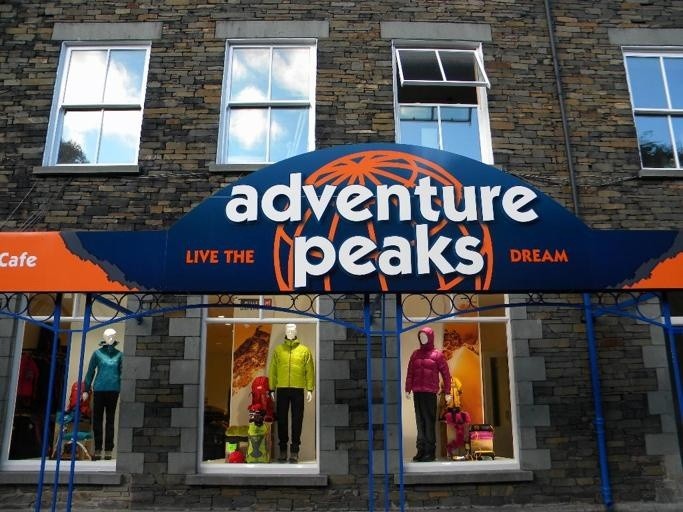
[250,375,276,423]
[67,380,92,416]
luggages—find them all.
[467,421,495,461]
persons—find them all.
[80,328,122,460]
[264,322,315,462]
[402,326,452,462]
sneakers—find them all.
[412,451,436,462]
[93,450,112,460]
[277,452,299,464]
